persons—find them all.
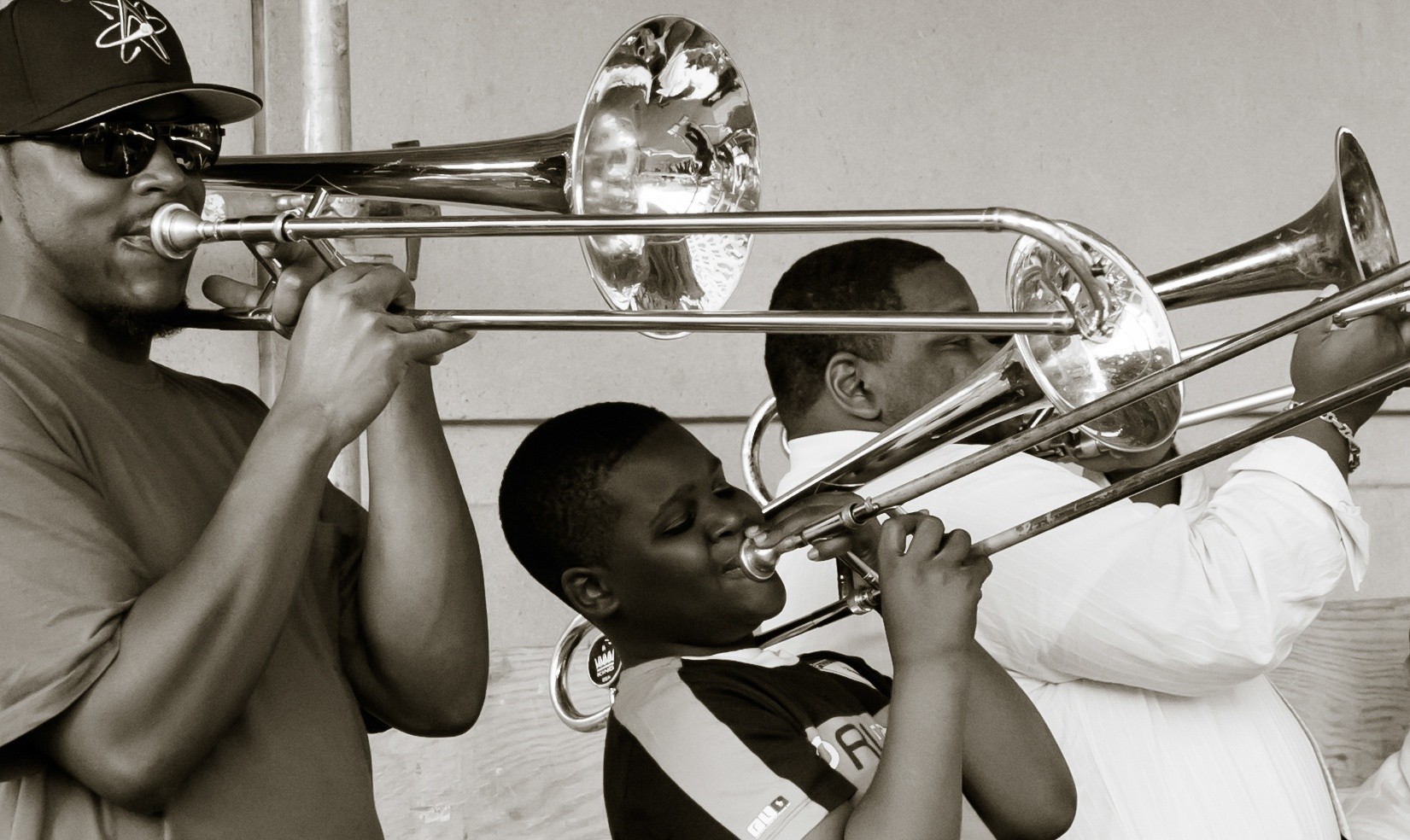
[497,401,1078,840]
[756,238,1410,840]
[0,0,488,840]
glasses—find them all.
[0,112,226,179]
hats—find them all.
[0,0,263,137]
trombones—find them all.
[147,17,1113,348]
[546,219,1410,734]
[740,125,1409,512]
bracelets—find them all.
[1281,400,1361,473]
